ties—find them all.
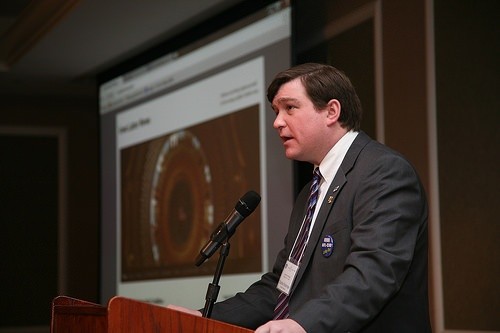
[273,167,324,321]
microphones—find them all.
[194,190,261,266]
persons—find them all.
[167,64,432,333]
